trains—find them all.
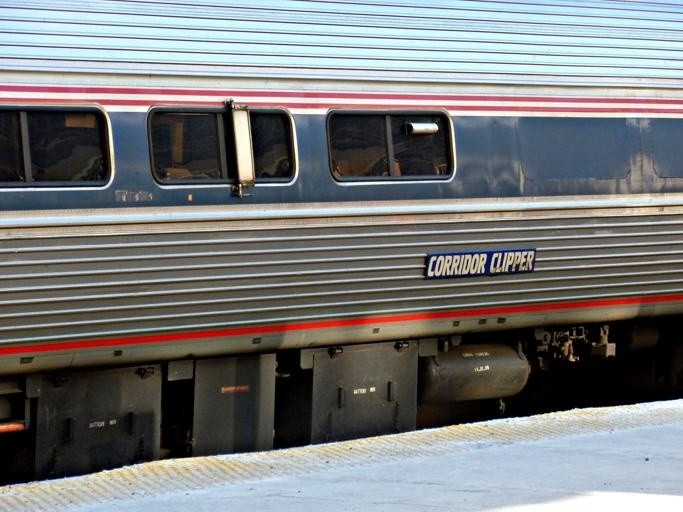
[1,0,683,486]
